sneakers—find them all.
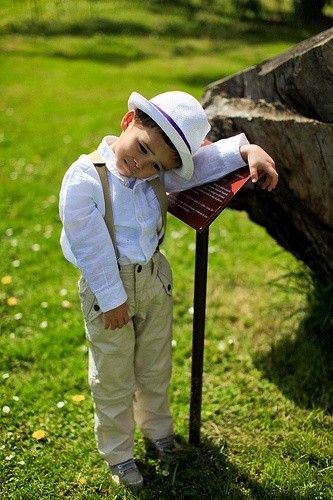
[110,458,144,489]
[146,434,177,455]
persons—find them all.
[58,90,278,493]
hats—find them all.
[128,91,211,181]
